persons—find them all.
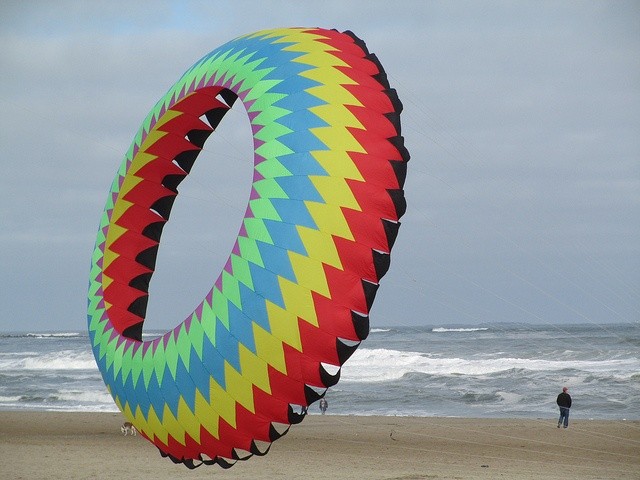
[319,396,328,415]
[556,385,577,429]
[299,404,309,416]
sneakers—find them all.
[564,425,567,428]
[557,423,561,428]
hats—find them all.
[563,387,568,392]
